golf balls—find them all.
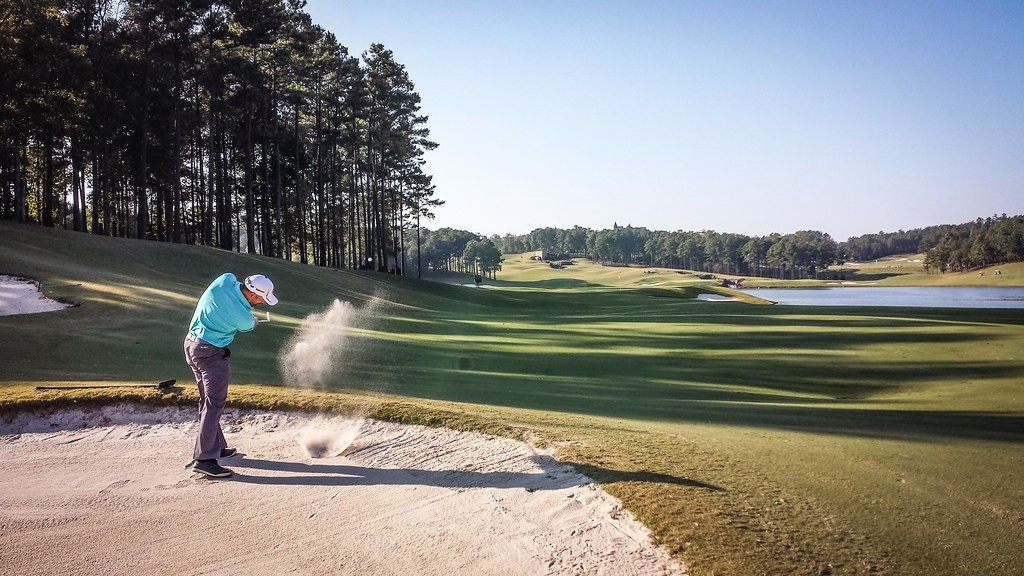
[368,257,373,262]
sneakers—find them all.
[220,448,237,458]
[191,458,231,477]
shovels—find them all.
[35,378,177,389]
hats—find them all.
[244,275,279,305]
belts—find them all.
[187,333,208,345]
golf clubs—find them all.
[257,311,271,323]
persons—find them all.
[184,272,279,477]
